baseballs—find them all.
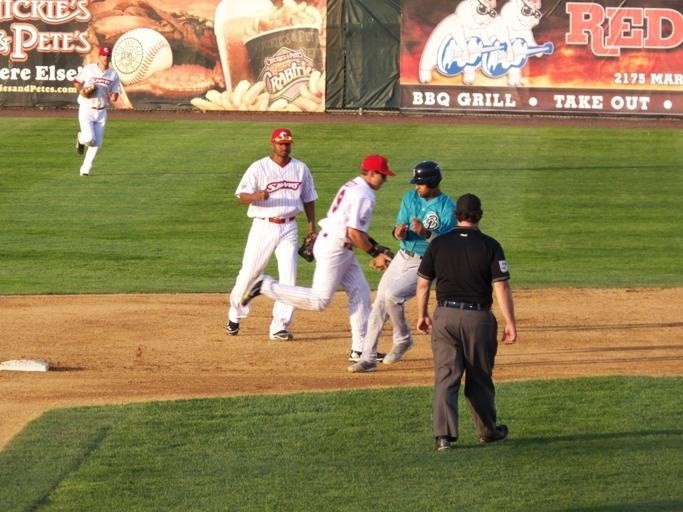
[111,28,173,85]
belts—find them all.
[402,248,422,258]
[270,217,295,223]
[440,301,488,311]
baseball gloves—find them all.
[298,232,315,262]
[370,250,394,271]
[83,85,95,98]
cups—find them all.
[244,25,323,106]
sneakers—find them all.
[435,437,450,450]
[228,320,239,335]
[241,275,263,305]
[77,142,85,155]
[480,425,508,444]
[271,331,292,340]
[349,343,409,372]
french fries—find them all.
[190,71,326,111]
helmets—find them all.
[410,162,441,184]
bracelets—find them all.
[392,228,397,241]
[368,237,378,245]
[368,247,379,257]
[424,230,431,239]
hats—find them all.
[272,128,294,144]
[457,193,480,212]
[362,156,394,176]
[99,47,112,55]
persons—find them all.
[72,46,120,176]
[225,128,318,341]
[241,155,395,363]
[416,194,518,450]
[348,161,459,372]
